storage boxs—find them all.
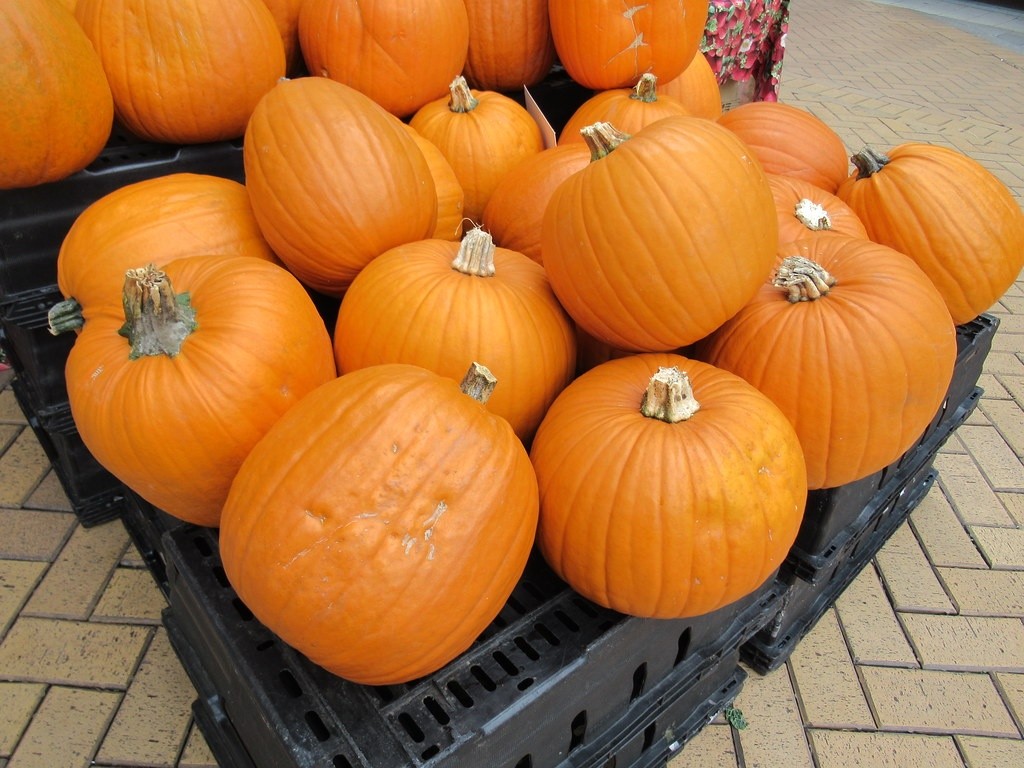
[0,55,1001,768]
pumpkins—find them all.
[0,0,1024,686]
